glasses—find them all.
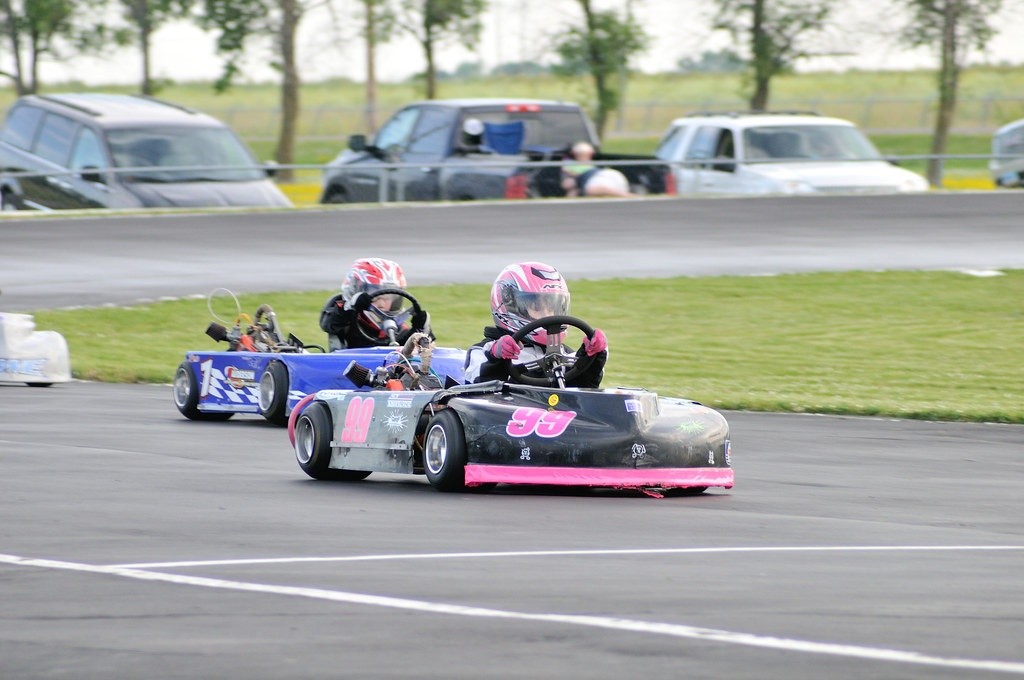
[519,297,557,312]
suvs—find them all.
[658,115,931,197]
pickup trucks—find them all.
[320,98,674,203]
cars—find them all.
[988,118,1024,189]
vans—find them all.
[0,91,294,211]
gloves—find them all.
[410,310,431,335]
[490,335,524,360]
[583,328,607,356]
[343,291,372,315]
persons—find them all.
[320,258,437,354]
[560,143,600,197]
[452,119,494,156]
[463,260,610,389]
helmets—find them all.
[491,262,571,345]
[341,257,407,329]
